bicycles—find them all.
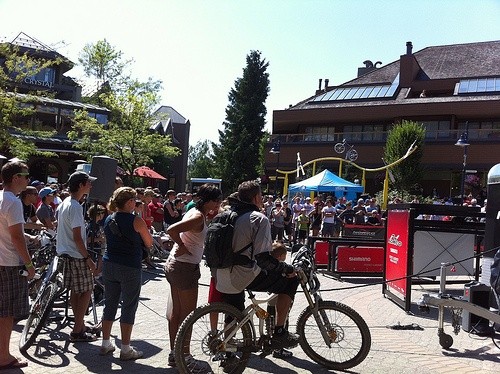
[17,230,151,352]
[173,244,371,374]
[334,137,359,162]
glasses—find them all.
[29,193,38,196]
[48,193,54,196]
[16,172,29,179]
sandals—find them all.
[86,325,101,333]
[69,331,97,341]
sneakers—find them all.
[119,346,144,361]
[270,331,301,352]
[99,343,115,355]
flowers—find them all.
[465,174,480,185]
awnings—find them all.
[133,165,166,180]
[117,167,127,176]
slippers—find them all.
[0,358,28,369]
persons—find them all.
[0,158,500,370]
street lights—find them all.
[454,120,470,207]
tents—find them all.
[289,169,364,203]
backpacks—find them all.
[205,207,257,269]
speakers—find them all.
[87,156,119,203]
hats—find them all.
[154,193,161,198]
[38,187,57,198]
[69,172,98,184]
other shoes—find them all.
[169,352,208,371]
[274,349,292,358]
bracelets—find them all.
[24,260,33,269]
[178,243,184,247]
[84,254,92,261]
